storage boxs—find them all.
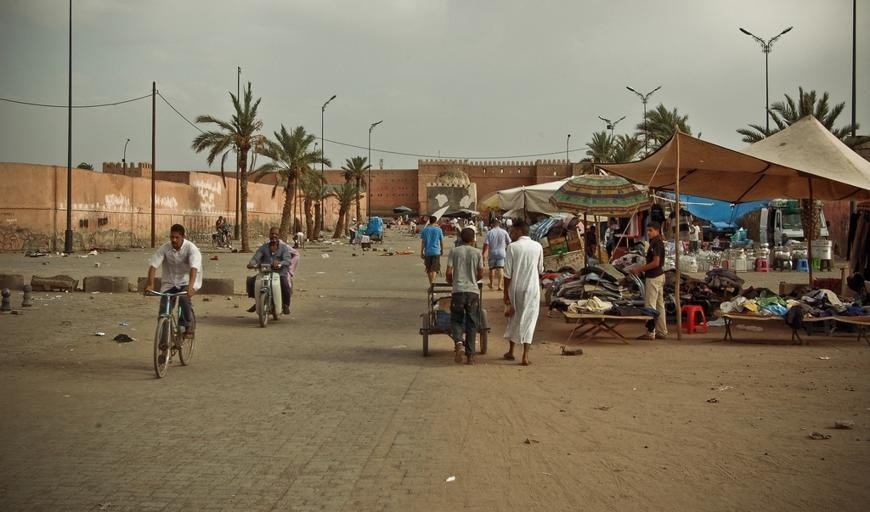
[539,229,582,256]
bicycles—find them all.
[143,286,197,379]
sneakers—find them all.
[245,303,257,313]
[635,335,667,340]
[281,305,291,315]
[466,355,476,365]
[454,344,465,364]
[186,322,196,339]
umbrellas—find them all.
[393,204,412,213]
[549,173,651,263]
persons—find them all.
[481,219,512,291]
[144,224,203,364]
[585,220,618,262]
[446,228,485,365]
[630,222,668,341]
[292,231,306,249]
[398,212,526,248]
[689,219,701,253]
[215,216,233,240]
[349,218,358,242]
[420,216,444,292]
[713,235,720,250]
[247,226,292,314]
[502,221,545,365]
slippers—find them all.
[504,352,514,360]
[521,358,531,366]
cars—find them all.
[691,216,745,243]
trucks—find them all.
[758,197,830,245]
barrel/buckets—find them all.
[811,240,832,260]
[367,216,383,236]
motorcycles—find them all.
[241,262,287,328]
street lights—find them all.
[320,93,339,229]
[625,85,664,155]
[597,114,628,164]
[566,133,572,162]
[367,119,386,220]
[123,138,130,176]
[312,142,319,170]
[736,25,795,138]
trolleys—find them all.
[418,276,490,358]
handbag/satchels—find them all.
[807,238,832,259]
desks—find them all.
[544,250,585,272]
[560,309,654,347]
[723,313,870,344]
[614,234,638,247]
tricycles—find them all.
[211,226,232,249]
[370,233,384,244]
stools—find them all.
[755,259,768,272]
[774,259,791,272]
[681,305,707,333]
[820,259,831,272]
[793,259,808,272]
